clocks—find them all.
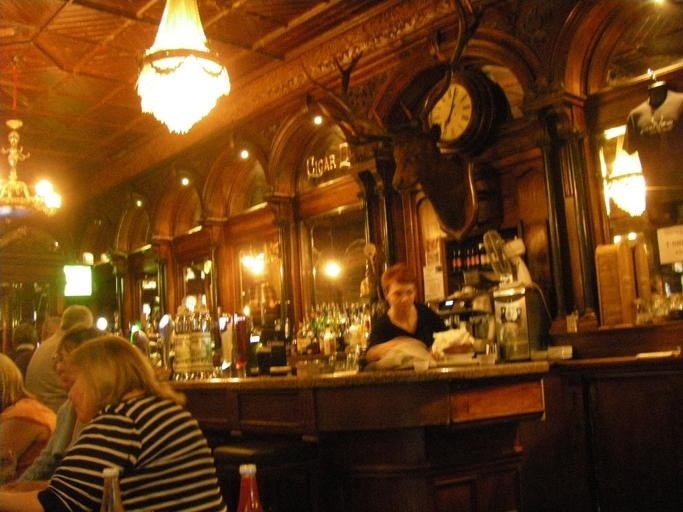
[424,72,496,156]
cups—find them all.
[304,352,359,370]
[475,339,498,365]
[411,351,431,372]
[255,347,270,377]
[630,292,683,327]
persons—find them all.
[622,78,682,204]
[1,303,232,511]
[364,261,448,360]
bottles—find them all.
[237,464,264,512]
[100,468,124,512]
[270,319,287,376]
[296,302,372,356]
[172,293,214,383]
[447,244,500,275]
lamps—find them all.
[0,120,60,217]
[135,0,231,135]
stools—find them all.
[213,441,298,512]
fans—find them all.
[484,230,532,288]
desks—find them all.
[548,357,683,511]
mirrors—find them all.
[1,229,70,351]
[225,155,283,348]
[129,214,166,327]
[172,189,213,319]
[586,0,683,321]
[294,121,369,314]
